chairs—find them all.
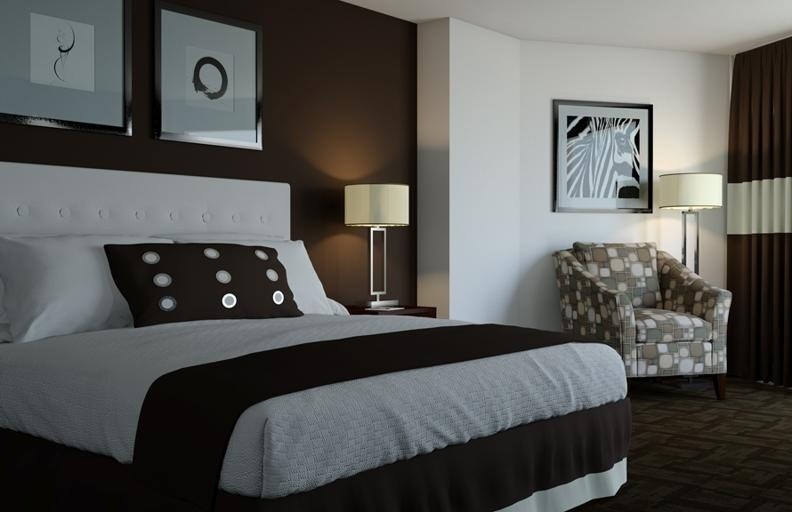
[553,241,732,401]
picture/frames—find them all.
[152,2,264,151]
[0,0,134,138]
[551,99,653,213]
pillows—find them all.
[1,233,176,344]
[151,236,333,316]
[104,242,304,328]
[572,242,664,309]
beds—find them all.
[1,160,631,512]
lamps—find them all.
[343,183,409,308]
[658,174,722,275]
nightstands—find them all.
[347,305,437,317]
